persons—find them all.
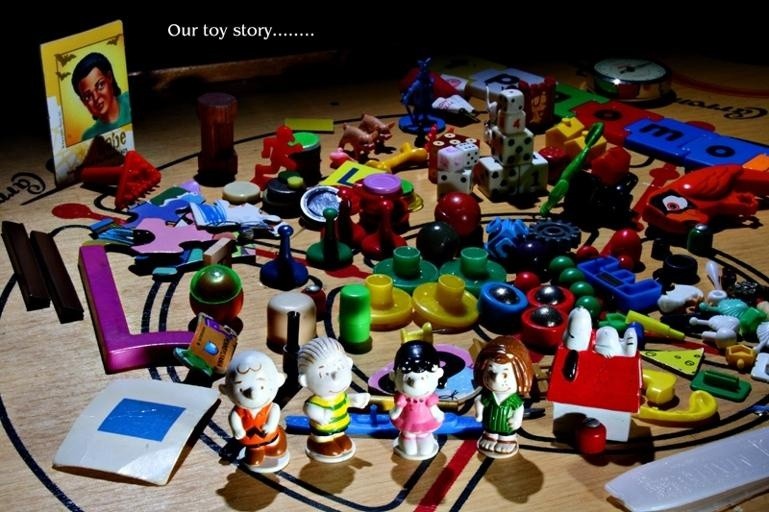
[77,58,769,512]
[71,51,131,142]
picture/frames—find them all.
[39,18,136,189]
